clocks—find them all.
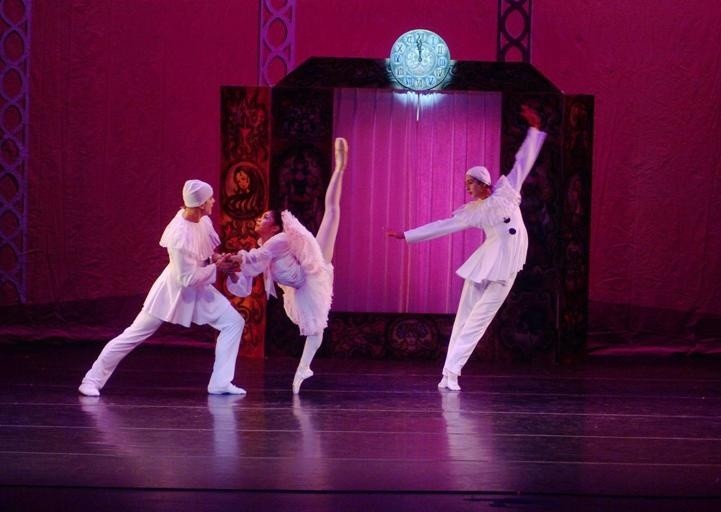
[390,28,453,93]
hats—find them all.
[467,165,492,186]
[182,179,213,206]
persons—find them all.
[233,166,256,195]
[442,389,480,491]
[78,180,247,396]
[292,394,322,489]
[218,138,349,395]
[387,104,549,391]
[78,394,244,487]
